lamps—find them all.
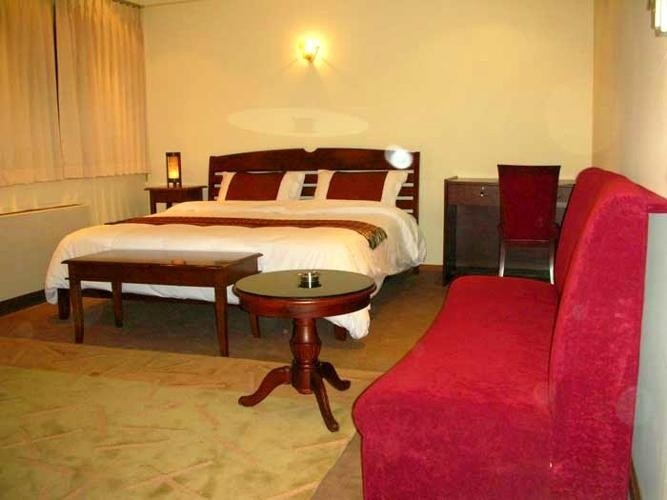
[166,152,181,188]
[302,41,320,65]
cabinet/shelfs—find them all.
[443,176,575,287]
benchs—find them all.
[59,250,261,358]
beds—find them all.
[45,148,427,339]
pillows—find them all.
[314,169,409,209]
[217,171,305,202]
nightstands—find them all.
[144,186,207,213]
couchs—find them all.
[352,167,667,500]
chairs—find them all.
[497,164,560,285]
